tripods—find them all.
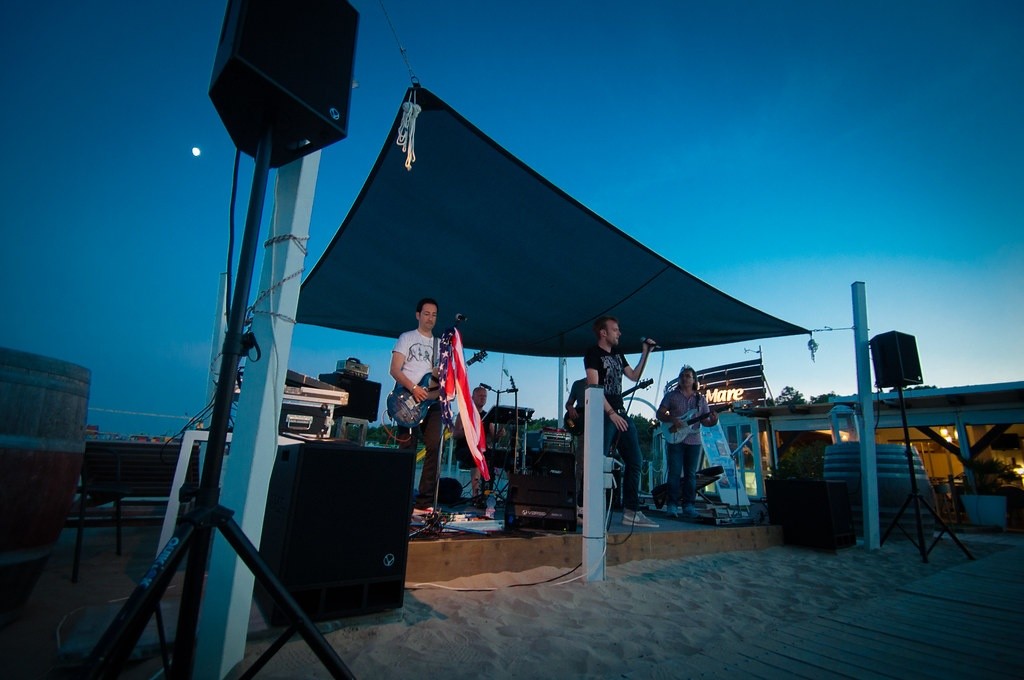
[879,385,974,564]
[79,130,355,680]
[409,320,492,540]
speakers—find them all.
[504,473,578,533]
[870,331,924,388]
[209,0,360,168]
[317,372,381,423]
[765,478,857,553]
[255,442,417,626]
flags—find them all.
[437,326,490,482]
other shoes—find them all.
[684,504,702,518]
[667,505,679,519]
[577,503,583,526]
[413,506,440,514]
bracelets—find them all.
[608,408,614,415]
[411,384,417,393]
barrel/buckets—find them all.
[0,347,92,626]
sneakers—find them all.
[621,508,661,527]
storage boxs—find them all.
[278,399,334,438]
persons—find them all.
[389,297,445,512]
[452,387,506,505]
[656,364,719,520]
[565,376,583,518]
[577,316,660,526]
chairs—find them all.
[72,446,124,585]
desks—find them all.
[157,430,304,561]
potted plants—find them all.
[960,458,1024,527]
[765,440,857,551]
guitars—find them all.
[563,378,654,436]
[386,350,488,430]
[661,398,754,445]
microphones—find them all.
[640,337,663,350]
[456,313,468,322]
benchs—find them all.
[75,443,200,527]
